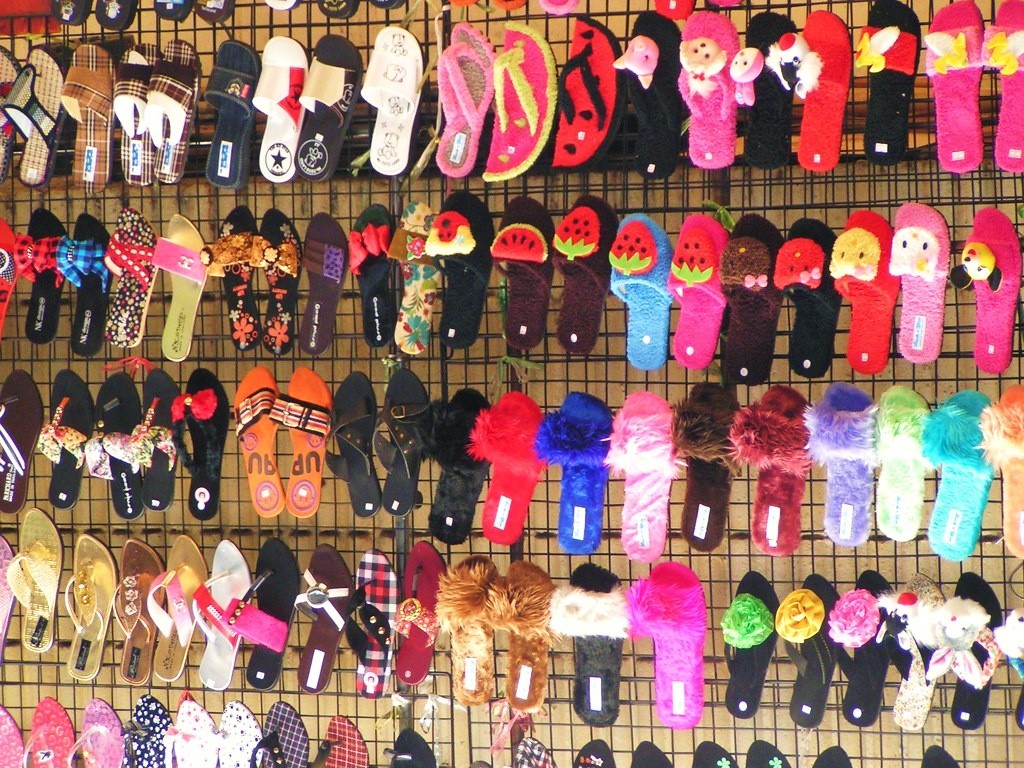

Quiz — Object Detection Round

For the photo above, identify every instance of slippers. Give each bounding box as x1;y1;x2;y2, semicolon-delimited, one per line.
0;0;1024;768
